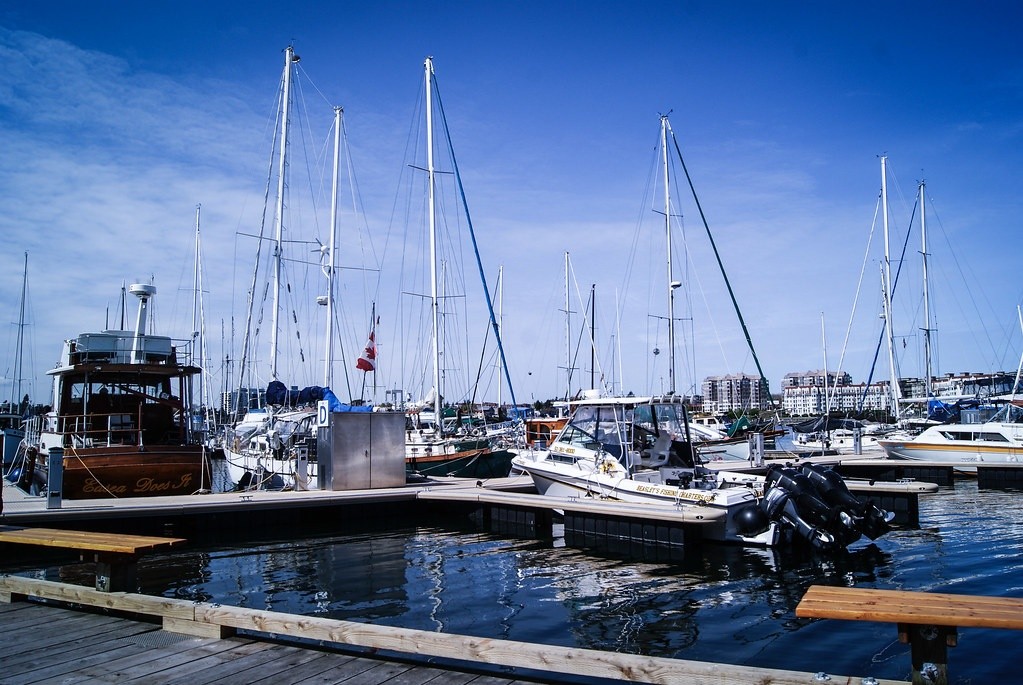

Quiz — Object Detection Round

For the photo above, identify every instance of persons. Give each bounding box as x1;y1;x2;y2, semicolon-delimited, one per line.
95;388;113;414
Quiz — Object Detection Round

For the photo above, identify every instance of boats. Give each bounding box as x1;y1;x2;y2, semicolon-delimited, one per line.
0;39;1022;579
